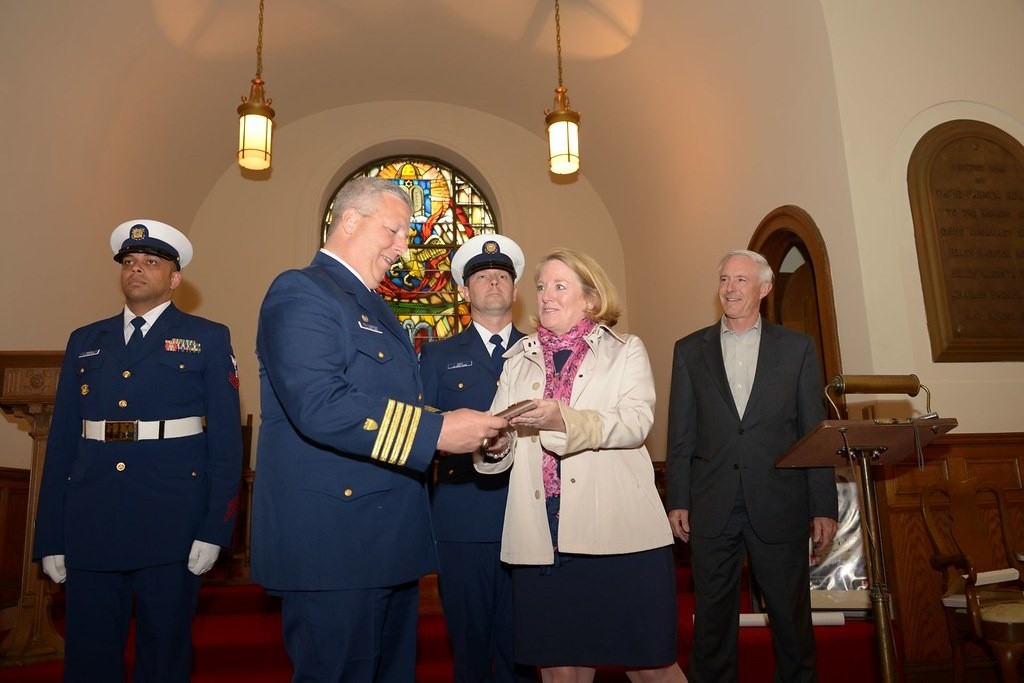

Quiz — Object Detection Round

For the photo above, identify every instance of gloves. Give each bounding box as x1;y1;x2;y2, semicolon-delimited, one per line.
187;540;220;575
42;555;67;584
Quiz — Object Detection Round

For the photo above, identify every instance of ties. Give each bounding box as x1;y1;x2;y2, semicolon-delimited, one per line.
127;316;147;353
489;334;506;374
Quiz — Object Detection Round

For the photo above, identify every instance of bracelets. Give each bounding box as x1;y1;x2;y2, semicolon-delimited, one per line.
486;433;511;459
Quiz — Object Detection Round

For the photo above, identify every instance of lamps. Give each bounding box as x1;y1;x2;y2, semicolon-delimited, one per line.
541;0;583;174
236;0;276;171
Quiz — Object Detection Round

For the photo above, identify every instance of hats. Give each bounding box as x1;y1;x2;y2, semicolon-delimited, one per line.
110;220;193;272
450;234;525;290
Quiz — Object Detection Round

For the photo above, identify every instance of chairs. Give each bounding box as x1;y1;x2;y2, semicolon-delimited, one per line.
919;479;1024;683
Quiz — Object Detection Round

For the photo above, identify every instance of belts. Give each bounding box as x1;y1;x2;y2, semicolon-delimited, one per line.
81;416;203;443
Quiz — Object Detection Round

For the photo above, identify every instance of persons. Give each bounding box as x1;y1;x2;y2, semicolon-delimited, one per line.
473;247;690;683
249;176;505;683
418;232;540;683
663;248;838;683
29;219;245;683
